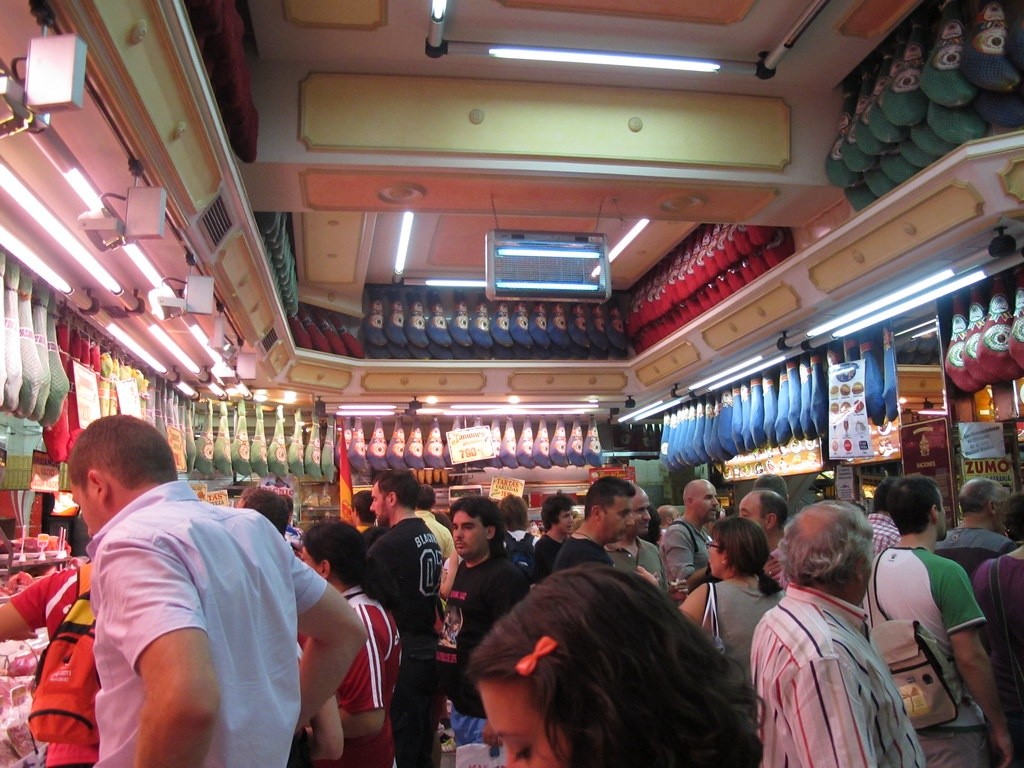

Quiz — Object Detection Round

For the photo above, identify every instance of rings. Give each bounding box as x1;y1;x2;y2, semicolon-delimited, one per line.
769;570;772;575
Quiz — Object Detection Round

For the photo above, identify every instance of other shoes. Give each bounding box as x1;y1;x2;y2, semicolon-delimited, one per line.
439;731;456;752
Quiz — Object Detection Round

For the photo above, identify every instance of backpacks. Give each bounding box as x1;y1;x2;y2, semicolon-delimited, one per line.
28;561;102;747
504;531;536;583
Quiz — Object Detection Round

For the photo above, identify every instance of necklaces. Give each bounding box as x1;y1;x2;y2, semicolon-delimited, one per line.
574;531;591;540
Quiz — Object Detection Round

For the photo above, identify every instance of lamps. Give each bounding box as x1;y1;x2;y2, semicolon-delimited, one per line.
617;224;1024;426
0;77;254;401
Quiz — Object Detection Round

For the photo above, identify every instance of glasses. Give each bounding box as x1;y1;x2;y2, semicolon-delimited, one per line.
705;540;720;550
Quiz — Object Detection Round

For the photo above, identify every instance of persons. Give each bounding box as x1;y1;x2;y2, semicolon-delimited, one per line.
658;475;1024;768
438;470;688;750
468;565;765;768
0;469;454;768
751;501;926;768
67;416;369;768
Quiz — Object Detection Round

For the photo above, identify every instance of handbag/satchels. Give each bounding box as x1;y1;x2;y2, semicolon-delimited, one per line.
868;618;959;728
455;743;505;768
